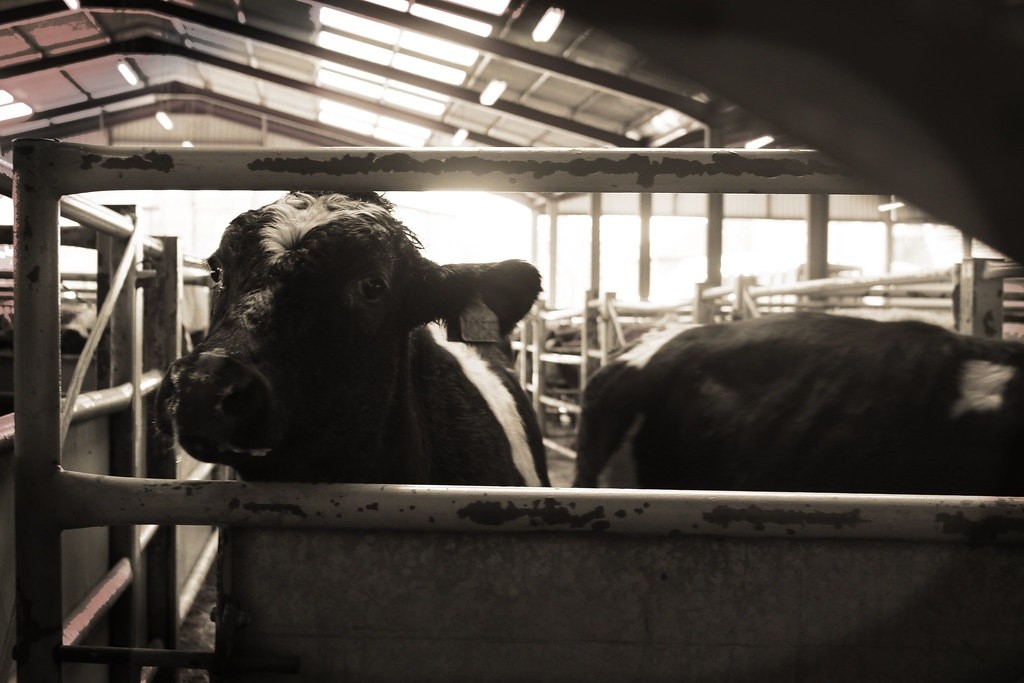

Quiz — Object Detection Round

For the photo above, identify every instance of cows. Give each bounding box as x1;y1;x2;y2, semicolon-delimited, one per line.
569;312;1024;496
152;190;550;488
